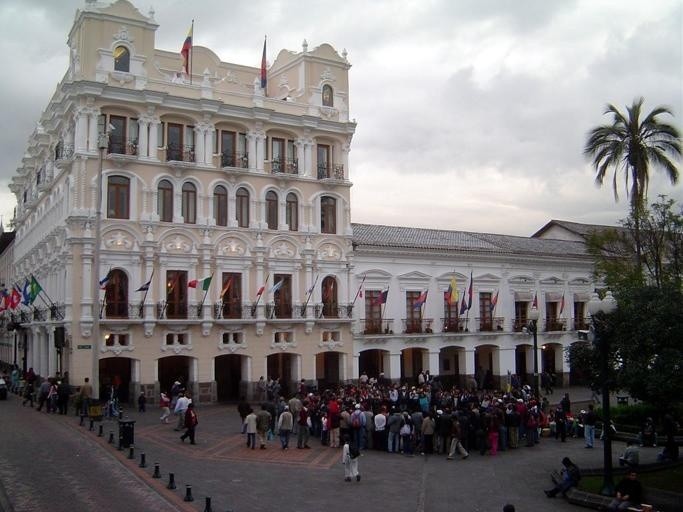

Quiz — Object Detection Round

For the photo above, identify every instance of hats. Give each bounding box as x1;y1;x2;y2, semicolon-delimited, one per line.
437;410;443;415
517;399;523;403
175;381;181;386
355;404;361;409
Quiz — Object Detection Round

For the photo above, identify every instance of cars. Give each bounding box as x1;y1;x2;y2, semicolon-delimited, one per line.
0;375;7;397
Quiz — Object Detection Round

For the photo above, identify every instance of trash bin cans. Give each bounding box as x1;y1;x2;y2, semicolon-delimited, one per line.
617;396;629;406
120;419;136;448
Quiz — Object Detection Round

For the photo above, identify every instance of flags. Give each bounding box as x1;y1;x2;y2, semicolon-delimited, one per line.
490;294;497;309
561;296;564;314
460;273;472;314
260;43;267;87
169;277;174;294
359;287;363;297
412;290;427;309
256;287;264;295
219;280;230;297
268;281;282;291
181;25;191;73
325;283;333;302
136;282;149;291
377;289;388;304
0;276;41;312
188;277;211;290
445;275;458;305
99;272;109;290
305;286;313;295
532;295;537;308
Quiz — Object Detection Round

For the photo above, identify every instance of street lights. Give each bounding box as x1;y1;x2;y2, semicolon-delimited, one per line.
586;287;618;497
528;304;541;411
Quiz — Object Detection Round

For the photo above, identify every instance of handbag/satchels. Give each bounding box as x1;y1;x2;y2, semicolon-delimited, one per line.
240;424;247;434
349;443;360;459
400;424;411;436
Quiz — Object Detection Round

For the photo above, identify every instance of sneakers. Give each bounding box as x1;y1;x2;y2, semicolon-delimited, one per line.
260;442;267;449
653;443;657;448
639;444;644;447
585;445;593;449
544;490;553;498
359;439;540;461
22;400;88;416
174;428;180;431
298;439;340;449
190;441;196;445
356;475;360;481
283;444;288;449
180;436;184;442
344;477;351;482
247;442;250;447
160;417;164;425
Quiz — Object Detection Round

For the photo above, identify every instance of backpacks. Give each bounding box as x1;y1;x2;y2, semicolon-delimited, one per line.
351;413;362;428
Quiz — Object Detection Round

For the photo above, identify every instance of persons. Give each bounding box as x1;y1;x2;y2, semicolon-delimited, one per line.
137;392;146;413
1;366;120;417
236;369;570;462
340;434;360;481
543;404;682;511
159;376;198;446
